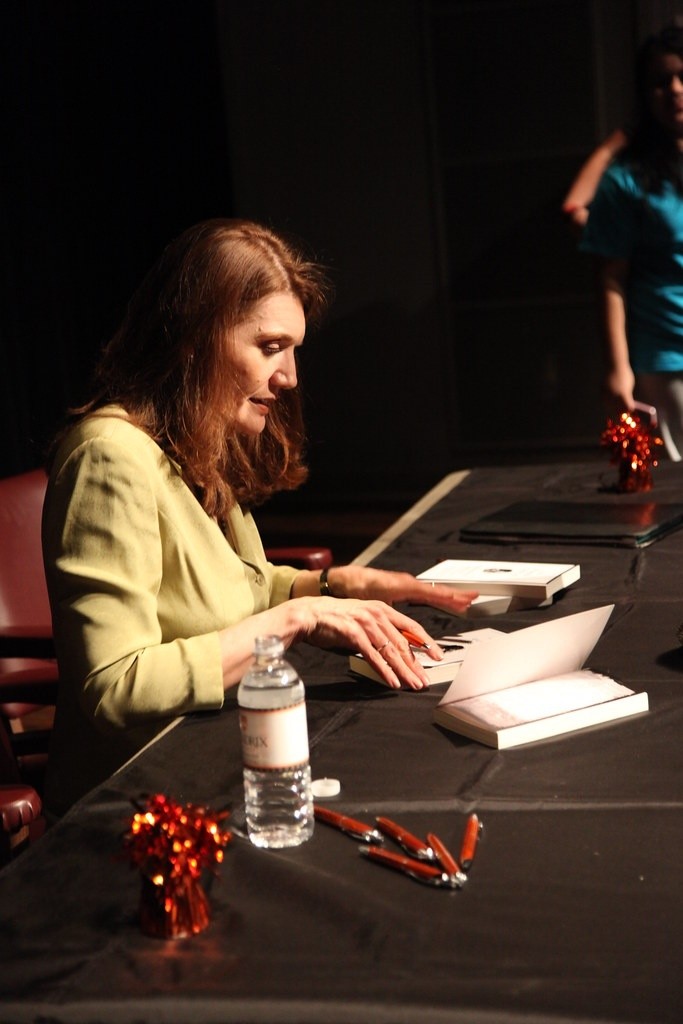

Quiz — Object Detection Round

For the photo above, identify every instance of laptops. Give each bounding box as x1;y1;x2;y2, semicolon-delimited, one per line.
459;501;683;548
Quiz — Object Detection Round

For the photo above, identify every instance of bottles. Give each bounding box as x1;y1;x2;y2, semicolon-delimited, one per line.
237;635;314;849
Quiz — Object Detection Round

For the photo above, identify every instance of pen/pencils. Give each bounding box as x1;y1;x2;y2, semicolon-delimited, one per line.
427;828;469;888
359;844;449;886
375;815;434;862
398;627;431;652
312;795;387;845
459;812;484;871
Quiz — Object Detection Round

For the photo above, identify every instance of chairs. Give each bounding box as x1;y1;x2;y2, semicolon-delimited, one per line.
0;468;332;875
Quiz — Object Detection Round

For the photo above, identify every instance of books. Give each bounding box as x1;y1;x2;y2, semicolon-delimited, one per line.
416;559;580;618
349;628;508;689
434;604;648;750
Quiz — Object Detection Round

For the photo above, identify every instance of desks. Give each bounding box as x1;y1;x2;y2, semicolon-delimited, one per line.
0;446;683;1024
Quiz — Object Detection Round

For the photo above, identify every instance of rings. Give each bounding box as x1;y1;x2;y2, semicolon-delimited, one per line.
431;581;435;587
378;640;390;651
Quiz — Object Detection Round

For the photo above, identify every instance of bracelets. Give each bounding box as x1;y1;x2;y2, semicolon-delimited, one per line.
320;566;333;597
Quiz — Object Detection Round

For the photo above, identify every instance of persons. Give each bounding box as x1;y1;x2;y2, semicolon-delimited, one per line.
563;14;683;464
41;222;481;835
